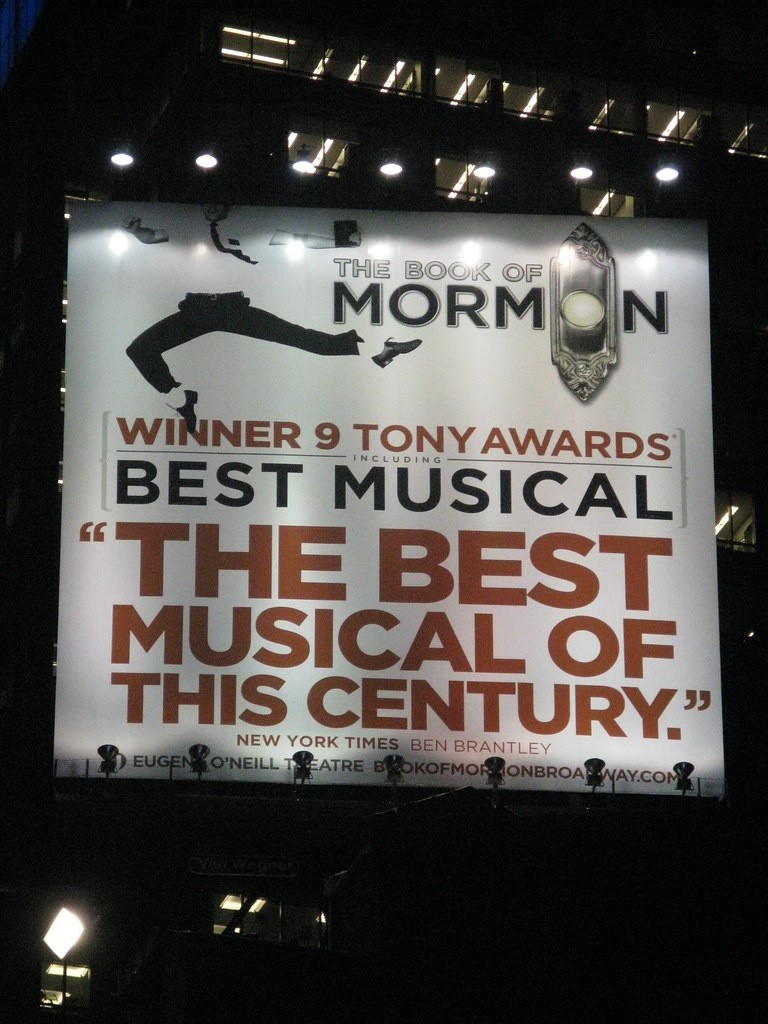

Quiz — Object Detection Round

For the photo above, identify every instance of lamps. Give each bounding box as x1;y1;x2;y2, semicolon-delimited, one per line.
673;761;694;795
383;754;405;786
189;744;211;781
292;751;314;784
484;757;506;789
96;744;119;778
584;758;606;793
292;143;315;172
